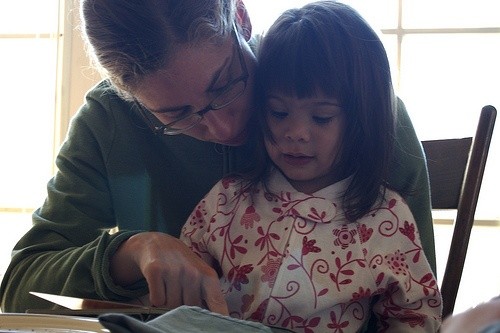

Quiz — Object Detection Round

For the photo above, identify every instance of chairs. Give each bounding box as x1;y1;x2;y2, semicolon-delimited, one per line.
421;105;497;322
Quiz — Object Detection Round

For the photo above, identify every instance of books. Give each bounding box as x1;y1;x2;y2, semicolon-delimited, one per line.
0;305;299;333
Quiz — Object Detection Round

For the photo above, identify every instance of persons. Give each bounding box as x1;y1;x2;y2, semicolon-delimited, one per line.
178;0;443;333
0;0;436;315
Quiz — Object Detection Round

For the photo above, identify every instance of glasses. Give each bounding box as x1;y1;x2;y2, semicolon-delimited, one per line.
133;19;249;136
